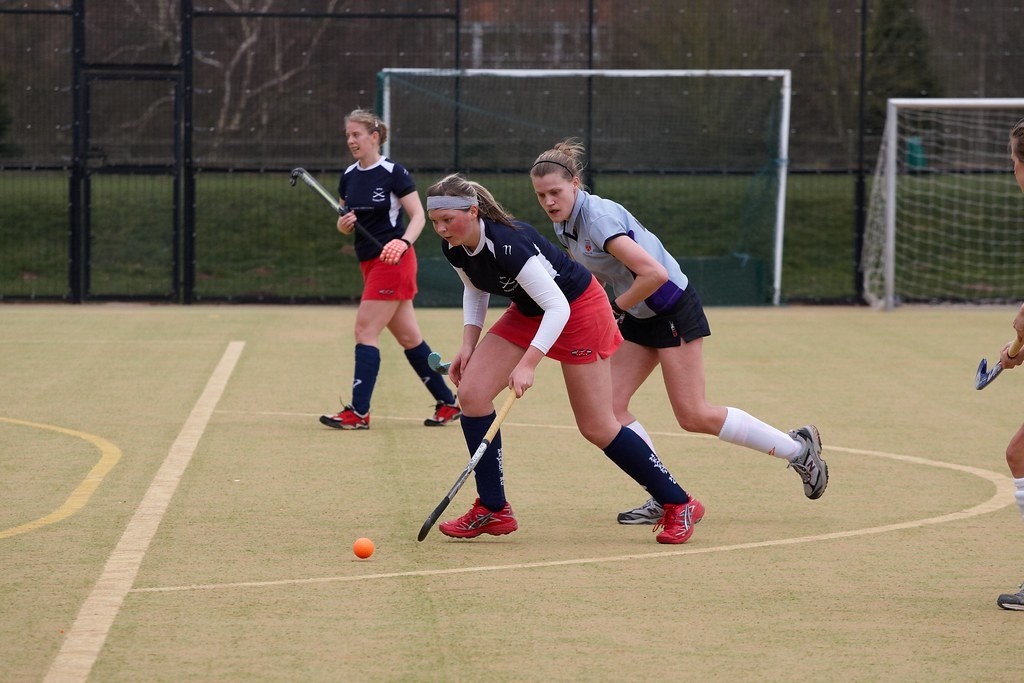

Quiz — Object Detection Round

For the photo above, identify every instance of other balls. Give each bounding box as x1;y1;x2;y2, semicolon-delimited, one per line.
352;536;376;560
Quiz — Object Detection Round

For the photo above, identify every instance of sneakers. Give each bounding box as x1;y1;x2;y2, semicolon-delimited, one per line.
424;394;463;426
997;582;1024;611
653;493;705;544
617;496;664;525
786;424;829;499
320;393;369;430
439;498;518;539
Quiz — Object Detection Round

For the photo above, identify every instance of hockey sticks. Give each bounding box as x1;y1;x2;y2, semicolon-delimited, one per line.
417;390;515;542
290;167;399;264
974;332;1024;391
427;351;453;375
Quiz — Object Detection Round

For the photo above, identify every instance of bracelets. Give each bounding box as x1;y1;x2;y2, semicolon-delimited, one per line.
610;300;624;314
399;238;410;248
614;312;621;319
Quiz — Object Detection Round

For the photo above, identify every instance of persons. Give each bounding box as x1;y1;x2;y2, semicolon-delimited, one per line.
997;118;1024;612
320;108;828;545
